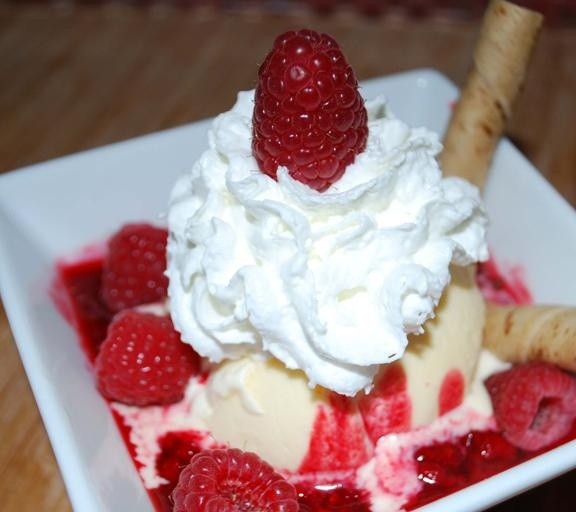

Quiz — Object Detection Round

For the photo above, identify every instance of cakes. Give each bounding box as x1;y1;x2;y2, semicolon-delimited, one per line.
67;0;576;512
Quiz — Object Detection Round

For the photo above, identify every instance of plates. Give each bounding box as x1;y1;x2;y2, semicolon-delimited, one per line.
1;64;575;512
